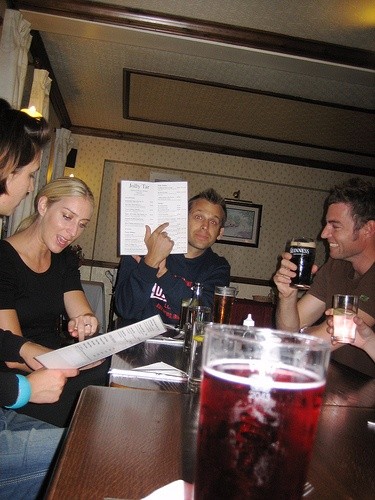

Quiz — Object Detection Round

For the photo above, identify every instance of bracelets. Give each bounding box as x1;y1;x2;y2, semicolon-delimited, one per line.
5;374;31;409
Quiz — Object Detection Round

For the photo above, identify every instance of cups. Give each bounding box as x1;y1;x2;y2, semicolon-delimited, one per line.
331;293;359;343
289;236;317;289
187;322;212;394
194;321;331;500
213;286;236;325
182;305;212;354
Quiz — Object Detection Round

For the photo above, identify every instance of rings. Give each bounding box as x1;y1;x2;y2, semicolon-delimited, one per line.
85;324;92;327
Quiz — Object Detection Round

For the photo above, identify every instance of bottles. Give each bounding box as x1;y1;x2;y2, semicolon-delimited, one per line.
241;313;255;352
185;281;203;348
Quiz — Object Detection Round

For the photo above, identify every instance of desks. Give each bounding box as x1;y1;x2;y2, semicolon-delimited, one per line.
40;318;375;500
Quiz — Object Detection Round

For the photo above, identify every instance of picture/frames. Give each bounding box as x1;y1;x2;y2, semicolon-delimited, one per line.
214;198;262;248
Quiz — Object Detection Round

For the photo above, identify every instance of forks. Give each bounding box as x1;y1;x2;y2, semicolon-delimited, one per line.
303;482;314;498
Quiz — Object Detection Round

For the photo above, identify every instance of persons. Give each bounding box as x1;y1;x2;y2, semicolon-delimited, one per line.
273;177;375;378
0;176;111;427
113;189;231;364
0;97;80;500
325;308;375;363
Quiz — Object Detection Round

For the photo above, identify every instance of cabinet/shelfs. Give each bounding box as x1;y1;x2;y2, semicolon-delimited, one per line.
229;298;274;328
